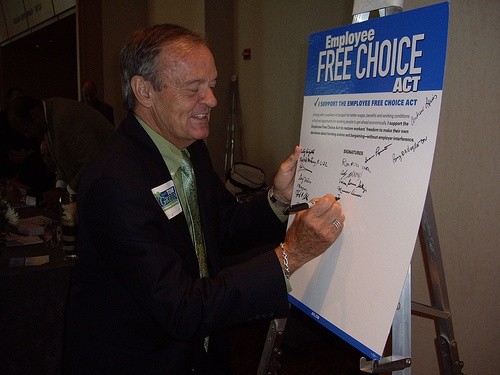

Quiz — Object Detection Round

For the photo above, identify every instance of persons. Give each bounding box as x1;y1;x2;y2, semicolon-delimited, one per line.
0;95;116;202
80;79;115;127
59;23;345;375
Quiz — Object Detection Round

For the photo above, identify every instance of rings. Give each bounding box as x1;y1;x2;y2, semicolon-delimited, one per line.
333;219;342;230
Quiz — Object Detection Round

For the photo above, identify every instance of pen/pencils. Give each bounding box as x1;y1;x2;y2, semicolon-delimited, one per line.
288;197;341;212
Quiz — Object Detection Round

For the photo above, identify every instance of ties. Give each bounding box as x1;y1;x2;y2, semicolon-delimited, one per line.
180;149;211;354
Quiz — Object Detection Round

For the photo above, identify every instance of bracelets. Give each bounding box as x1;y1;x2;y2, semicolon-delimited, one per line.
269;185;291;208
280;242;291;280
